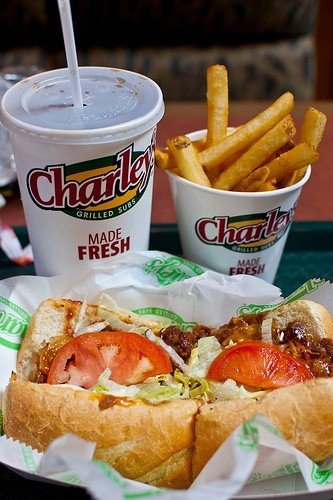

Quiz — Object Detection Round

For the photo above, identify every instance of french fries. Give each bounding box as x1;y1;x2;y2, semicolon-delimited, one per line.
156;63;326;192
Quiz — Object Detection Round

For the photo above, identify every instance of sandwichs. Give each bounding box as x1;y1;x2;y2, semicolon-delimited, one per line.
2;296;333;490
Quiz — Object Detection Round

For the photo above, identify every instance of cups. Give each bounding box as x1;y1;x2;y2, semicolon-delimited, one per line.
0;66;165;278
167;128;312;285
